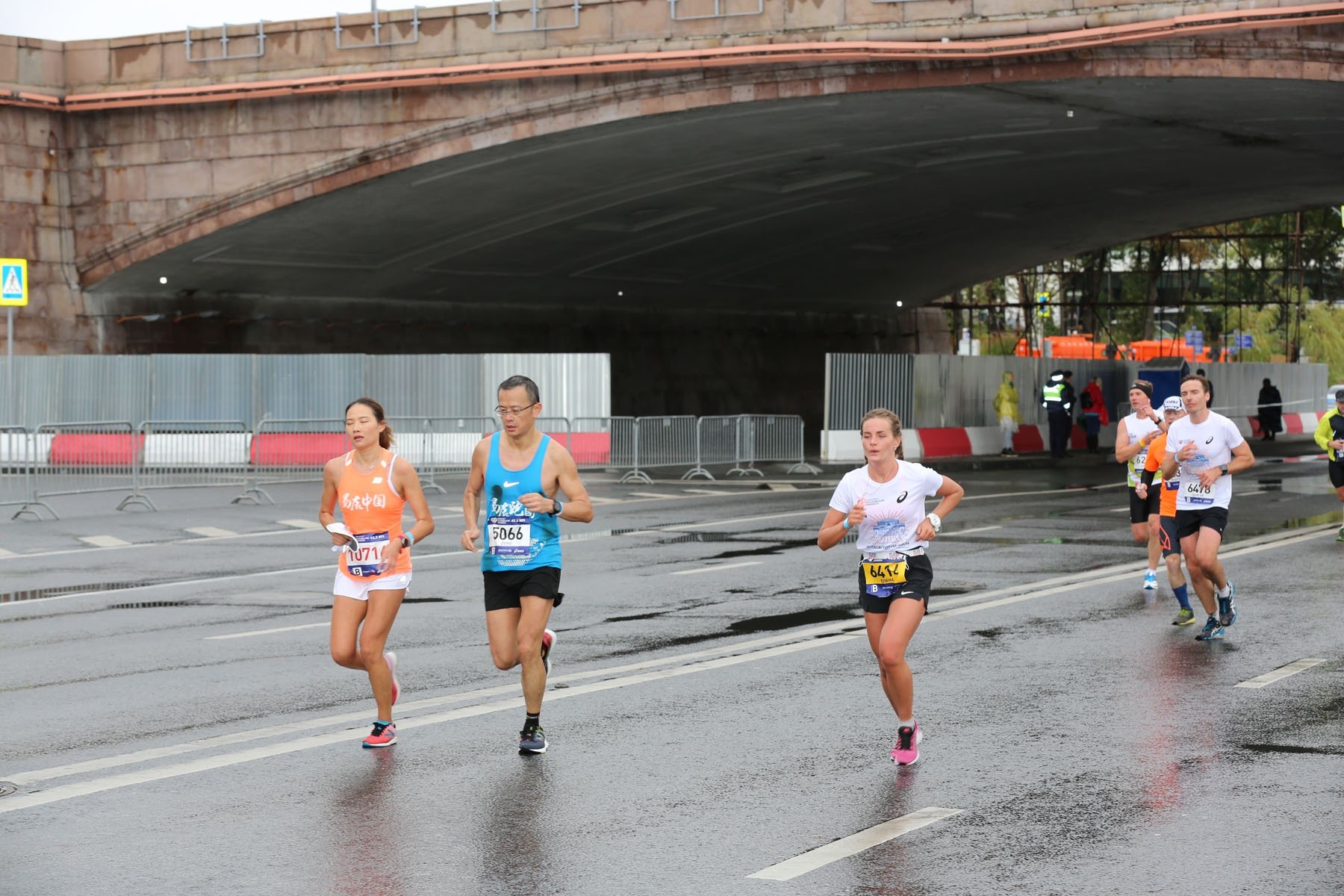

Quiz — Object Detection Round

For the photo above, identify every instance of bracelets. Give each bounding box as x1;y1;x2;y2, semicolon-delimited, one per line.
405;531;414;545
843;517;852;531
1154;417;1163;425
555;501;564;516
1174;454;1182;463
1139;438;1146;448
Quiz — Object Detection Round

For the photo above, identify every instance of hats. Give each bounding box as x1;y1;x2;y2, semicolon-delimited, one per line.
1130;379;1153;400
1163;395;1187;413
1335;389;1344;402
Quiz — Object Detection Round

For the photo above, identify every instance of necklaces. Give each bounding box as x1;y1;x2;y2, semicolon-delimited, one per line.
879;462;895;486
369;465;373;469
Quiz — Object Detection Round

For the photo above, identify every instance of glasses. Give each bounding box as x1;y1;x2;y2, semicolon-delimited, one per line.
494;403;537;416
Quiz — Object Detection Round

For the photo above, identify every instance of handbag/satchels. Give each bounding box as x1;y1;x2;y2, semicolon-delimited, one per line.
1099;405;1110;426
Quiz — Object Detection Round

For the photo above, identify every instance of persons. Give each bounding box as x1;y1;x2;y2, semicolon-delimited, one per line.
462;375;593;755
1135;368;1214;626
1314;389;1344;541
1115;379;1168;590
1258;378;1283;441
993;370;1109;458
319;397;434;747
1163;375;1255;641
818;407;965;765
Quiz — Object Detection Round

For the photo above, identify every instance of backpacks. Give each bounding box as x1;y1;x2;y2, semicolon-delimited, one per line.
1080;389;1093;409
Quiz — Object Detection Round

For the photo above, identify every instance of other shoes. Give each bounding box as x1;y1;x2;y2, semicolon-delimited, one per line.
1261;436;1276;442
1335;528;1344;541
1001;448;1018;458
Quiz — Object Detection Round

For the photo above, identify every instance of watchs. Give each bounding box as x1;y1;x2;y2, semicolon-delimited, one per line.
926;513;941;532
548;500;562;518
1219;465;1228;476
397;535;408;548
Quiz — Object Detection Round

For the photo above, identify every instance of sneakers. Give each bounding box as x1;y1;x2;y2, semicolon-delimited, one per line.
1143;569;1159;589
1216;581;1238;626
1171;608;1196;626
1195;617;1227;640
517;724;549;755
895;720;920;765
539;628;557;678
362;721;398;747
890;730;923;760
382;651;401;706
